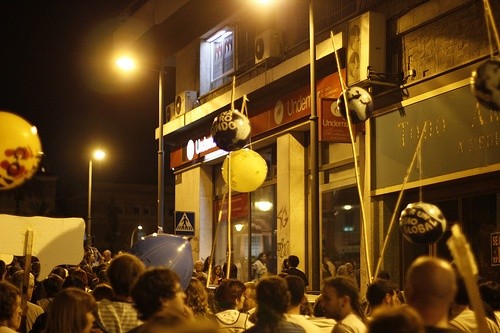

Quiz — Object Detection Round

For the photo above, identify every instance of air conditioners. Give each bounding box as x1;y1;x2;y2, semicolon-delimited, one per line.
165;102;175;123
254;29;281;66
175;91;197;118
346;11;386;88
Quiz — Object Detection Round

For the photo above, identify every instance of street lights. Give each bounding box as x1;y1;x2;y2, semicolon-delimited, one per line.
114;55;171;234
87;149;106;247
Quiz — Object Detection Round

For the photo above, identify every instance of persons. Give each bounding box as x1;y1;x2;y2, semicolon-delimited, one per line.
278;255;309;291
214;262;500;333
322;255;359;284
230;253;278;280
0;249;214;333
404;255;462;333
